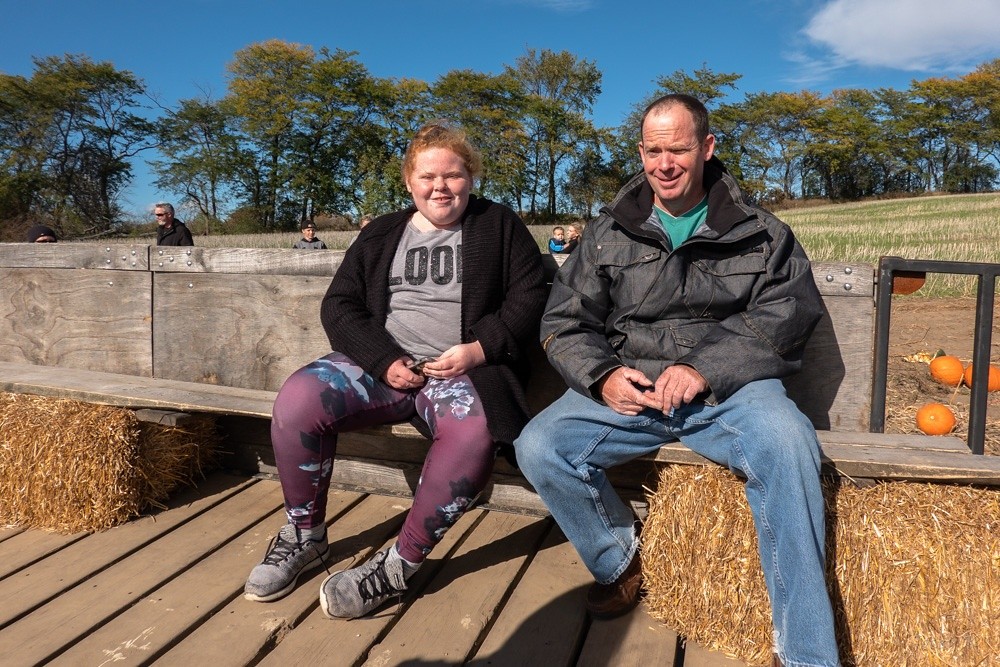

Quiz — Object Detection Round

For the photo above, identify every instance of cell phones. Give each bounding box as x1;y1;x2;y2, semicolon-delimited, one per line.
405;357;437;374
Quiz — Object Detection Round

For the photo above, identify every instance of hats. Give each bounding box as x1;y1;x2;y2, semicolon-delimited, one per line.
28;226;57;243
301;220;317;228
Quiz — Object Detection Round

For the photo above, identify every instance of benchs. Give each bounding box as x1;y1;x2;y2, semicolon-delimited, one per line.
0;239;1000;521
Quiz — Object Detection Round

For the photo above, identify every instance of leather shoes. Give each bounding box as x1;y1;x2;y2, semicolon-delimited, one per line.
586;550;641;617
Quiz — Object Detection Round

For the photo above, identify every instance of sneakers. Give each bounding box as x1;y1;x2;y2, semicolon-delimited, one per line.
244;524;330;602
320;548;408;619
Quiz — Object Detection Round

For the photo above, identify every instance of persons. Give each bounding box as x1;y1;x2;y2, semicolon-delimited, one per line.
29;226;57;243
155;203;194;246
243;126;559;620
562;222;584;254
512;94;843;667
548;226;570;254
349;216;372;249
293;220;328;250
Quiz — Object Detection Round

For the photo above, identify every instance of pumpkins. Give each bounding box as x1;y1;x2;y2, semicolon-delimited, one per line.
964;362;1000;392
930;356;965;387
916;403;957;435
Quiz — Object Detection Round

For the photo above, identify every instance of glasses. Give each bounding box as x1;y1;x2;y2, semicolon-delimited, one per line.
156;212;168;216
36;240;56;243
